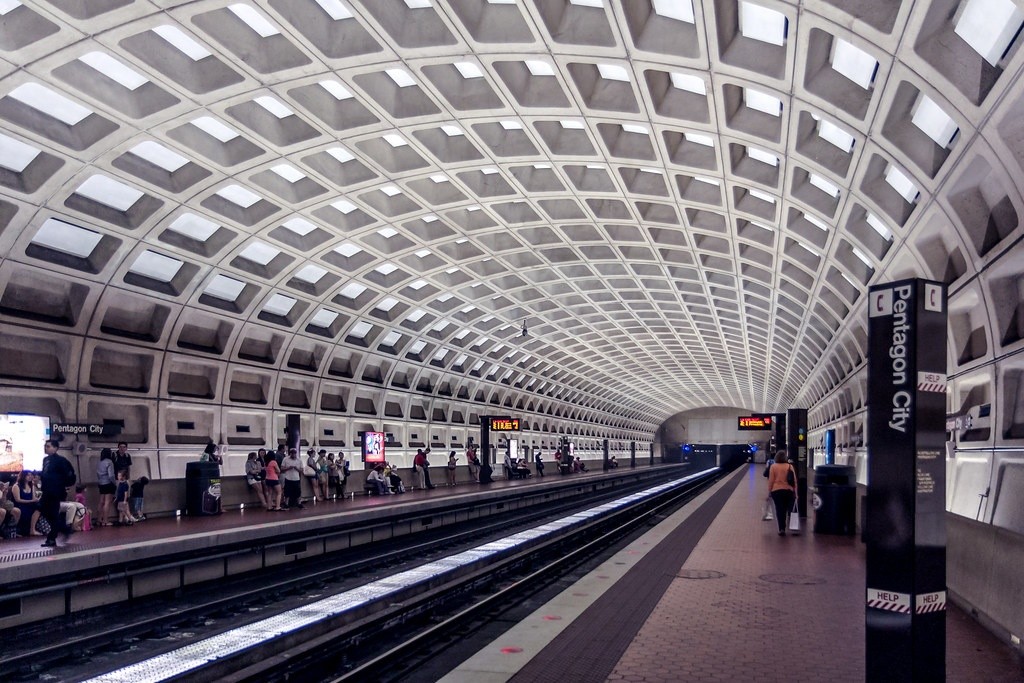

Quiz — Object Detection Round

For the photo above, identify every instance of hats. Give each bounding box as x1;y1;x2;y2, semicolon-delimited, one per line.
289;448;297;453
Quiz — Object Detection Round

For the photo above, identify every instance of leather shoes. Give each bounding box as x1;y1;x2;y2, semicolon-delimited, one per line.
62;524;72;541
41;541;57;547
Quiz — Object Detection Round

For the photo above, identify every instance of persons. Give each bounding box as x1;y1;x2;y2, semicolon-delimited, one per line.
504;451;532;480
467;445;495;484
535;452;545;477
414;448;436;490
200;443;350;511
763;450;799;536
555;449;589;474
609;457;618;469
0;439;149;547
448;450;460;486
367;461;406;496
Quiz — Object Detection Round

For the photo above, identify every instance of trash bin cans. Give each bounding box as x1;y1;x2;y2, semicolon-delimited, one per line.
813;464;858;536
185;462;222;516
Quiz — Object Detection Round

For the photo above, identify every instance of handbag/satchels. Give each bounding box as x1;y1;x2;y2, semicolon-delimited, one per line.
760;497;775;521
787;464;795;486
789;499;801;530
303;466;316;476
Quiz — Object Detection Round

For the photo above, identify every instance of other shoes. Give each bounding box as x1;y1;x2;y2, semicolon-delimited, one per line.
298;504;307;509
97;516;146;526
274;507;282;511
30;531;43;536
282;505;289;510
267;507;275;511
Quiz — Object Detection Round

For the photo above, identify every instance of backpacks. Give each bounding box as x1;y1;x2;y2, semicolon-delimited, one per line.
63;461;76;486
